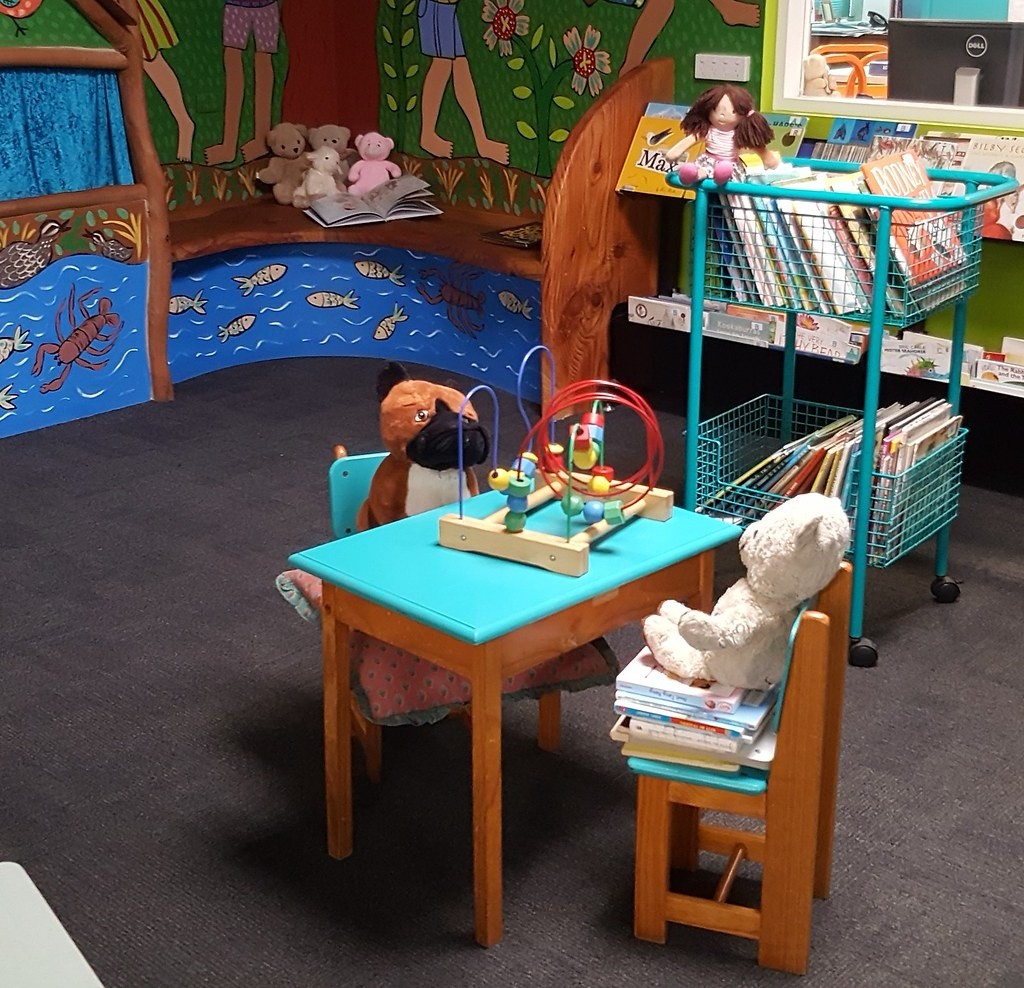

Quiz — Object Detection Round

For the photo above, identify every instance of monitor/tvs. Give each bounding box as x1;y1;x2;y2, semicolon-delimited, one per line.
888;18;1024;108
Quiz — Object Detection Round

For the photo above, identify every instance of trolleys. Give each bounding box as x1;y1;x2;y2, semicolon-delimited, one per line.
665;156;1020;667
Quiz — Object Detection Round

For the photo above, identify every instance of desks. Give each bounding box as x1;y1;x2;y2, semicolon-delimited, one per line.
285;470;741;951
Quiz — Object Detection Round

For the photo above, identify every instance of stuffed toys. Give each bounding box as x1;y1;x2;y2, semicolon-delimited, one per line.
804;55;840;99
640;489;856;685
664;85;777;184
357;361;487;533
256;120;403;209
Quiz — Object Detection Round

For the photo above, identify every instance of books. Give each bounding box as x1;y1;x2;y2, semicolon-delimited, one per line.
617;101;705;201
709;150;966;324
302;171;444;228
696;394;967;556
607;645;791;758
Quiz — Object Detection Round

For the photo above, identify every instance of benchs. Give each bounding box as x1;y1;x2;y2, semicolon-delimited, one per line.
153;190;562;415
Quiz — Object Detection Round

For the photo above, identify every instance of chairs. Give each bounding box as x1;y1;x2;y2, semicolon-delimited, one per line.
331;439;562;785
628;557;852;977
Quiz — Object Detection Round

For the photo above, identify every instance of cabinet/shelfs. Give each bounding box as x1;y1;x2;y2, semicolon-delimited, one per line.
664;150;1019;668
